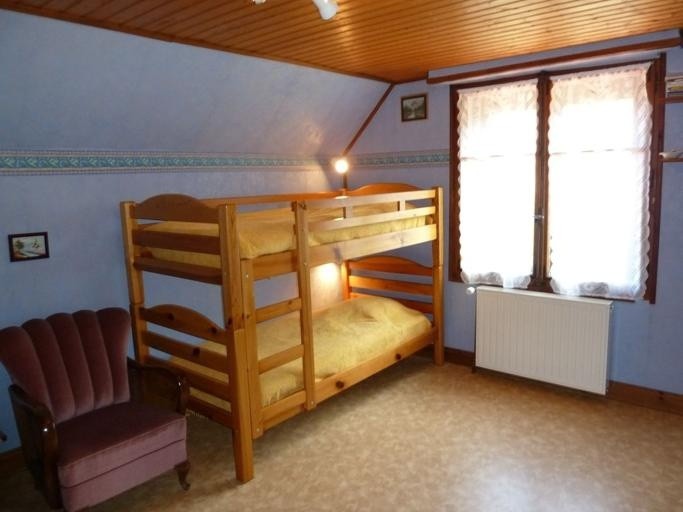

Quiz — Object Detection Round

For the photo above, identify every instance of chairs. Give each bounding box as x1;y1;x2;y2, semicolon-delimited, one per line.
0;306;194;511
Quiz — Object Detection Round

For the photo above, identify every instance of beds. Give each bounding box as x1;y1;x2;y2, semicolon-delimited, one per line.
118;180;446;485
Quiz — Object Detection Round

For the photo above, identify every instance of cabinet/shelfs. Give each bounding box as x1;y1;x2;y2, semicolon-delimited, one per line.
653;94;682;163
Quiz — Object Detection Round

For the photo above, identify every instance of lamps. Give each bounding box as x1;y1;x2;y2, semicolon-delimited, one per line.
251;0;340;21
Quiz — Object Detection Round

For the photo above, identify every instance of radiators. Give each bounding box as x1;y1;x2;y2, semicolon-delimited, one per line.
473;284;616;398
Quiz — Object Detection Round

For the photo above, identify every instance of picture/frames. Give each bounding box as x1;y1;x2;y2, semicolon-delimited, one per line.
7;231;51;263
399;91;429;122
